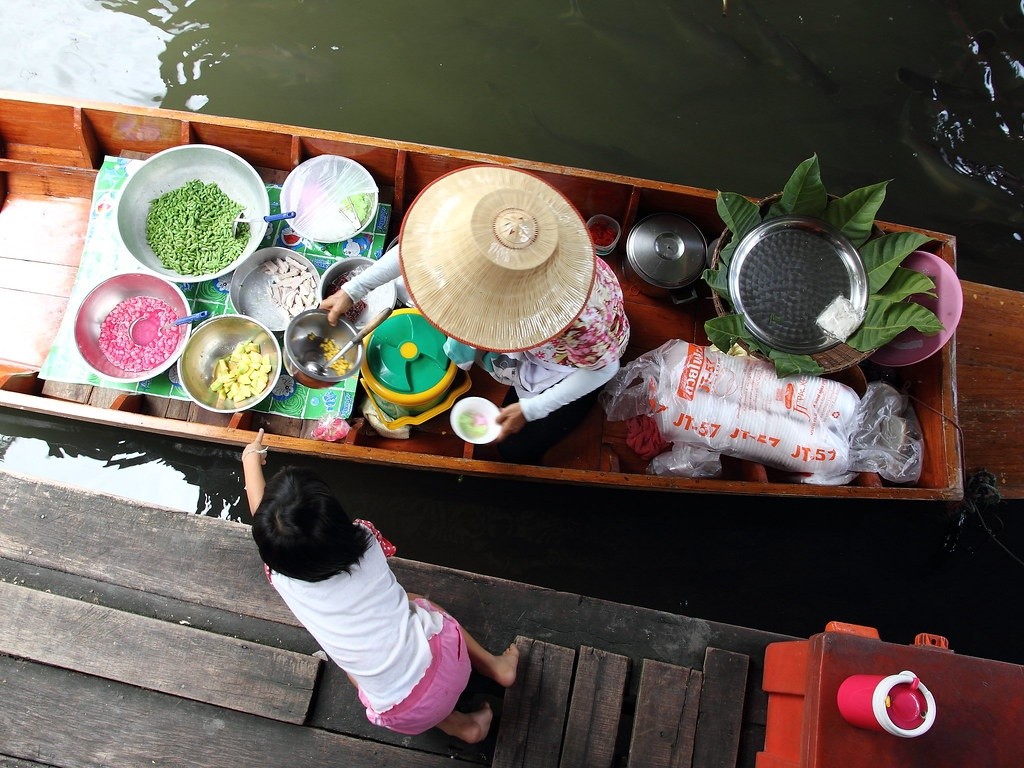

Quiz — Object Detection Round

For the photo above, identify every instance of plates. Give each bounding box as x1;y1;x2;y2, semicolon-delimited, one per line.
449;396;503;444
280;156;379;243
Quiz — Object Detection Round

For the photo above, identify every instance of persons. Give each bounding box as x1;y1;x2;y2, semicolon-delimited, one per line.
242;429;519;742
318;165;629;443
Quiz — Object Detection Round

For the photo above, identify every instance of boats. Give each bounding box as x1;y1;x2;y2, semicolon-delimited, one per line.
0;91;1024;509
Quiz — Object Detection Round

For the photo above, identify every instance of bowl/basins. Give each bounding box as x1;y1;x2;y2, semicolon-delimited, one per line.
585;215;620;255
117;145;270;284
74;272;191;383
176;314;283;413
229;248;321;332
871;251;963;368
320;258;397;329
654;341;859;473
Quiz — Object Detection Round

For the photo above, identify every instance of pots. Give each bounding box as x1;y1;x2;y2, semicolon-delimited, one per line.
282;310;364;388
624;211;708;306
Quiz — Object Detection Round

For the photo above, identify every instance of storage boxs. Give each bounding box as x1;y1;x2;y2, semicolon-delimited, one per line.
756;621;1024;768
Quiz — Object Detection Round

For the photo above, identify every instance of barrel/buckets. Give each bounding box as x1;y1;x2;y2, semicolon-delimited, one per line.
360;307;472;430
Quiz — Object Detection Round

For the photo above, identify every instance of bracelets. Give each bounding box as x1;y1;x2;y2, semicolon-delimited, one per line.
242;447;269;459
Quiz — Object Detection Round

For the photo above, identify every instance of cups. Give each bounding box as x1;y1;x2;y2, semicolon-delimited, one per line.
837;672;936;739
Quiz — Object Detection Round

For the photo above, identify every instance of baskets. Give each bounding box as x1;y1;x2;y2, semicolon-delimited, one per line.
710;191;907;375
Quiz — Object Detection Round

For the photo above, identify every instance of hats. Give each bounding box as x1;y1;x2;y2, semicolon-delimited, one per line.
397;165;597;353
